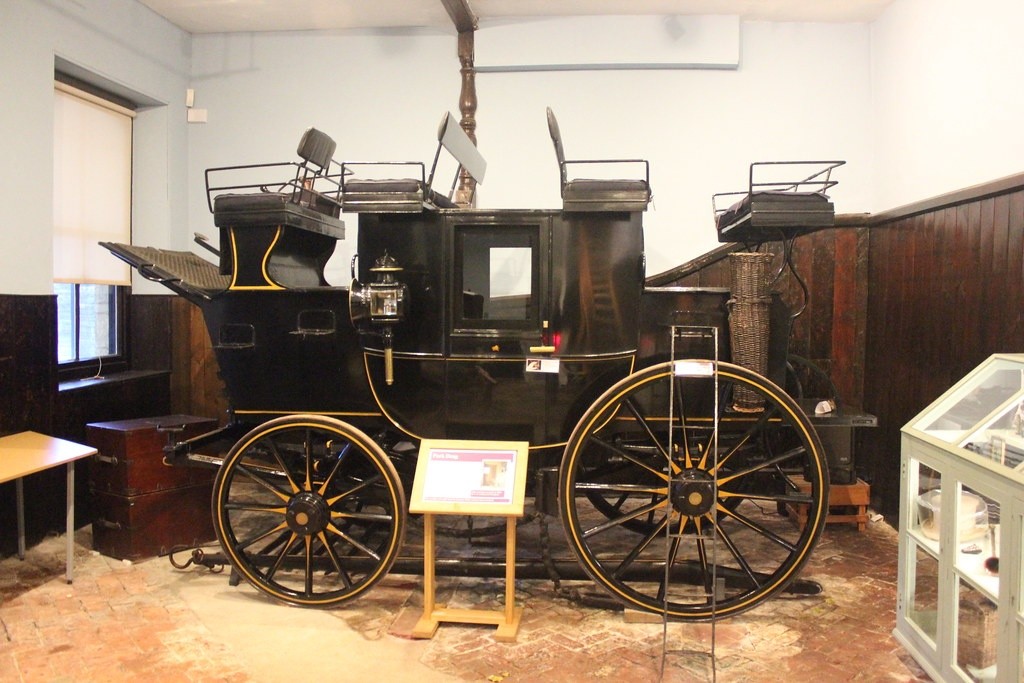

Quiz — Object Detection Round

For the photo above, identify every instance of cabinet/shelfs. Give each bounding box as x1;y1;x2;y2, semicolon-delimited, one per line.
900;352;1024;683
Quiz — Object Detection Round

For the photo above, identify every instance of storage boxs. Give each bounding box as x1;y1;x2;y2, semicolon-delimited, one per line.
917;487;989;541
89;484;218;565
86;413;220;494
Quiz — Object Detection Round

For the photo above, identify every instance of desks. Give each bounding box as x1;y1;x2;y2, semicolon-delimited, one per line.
0;429;99;584
786;474;871;532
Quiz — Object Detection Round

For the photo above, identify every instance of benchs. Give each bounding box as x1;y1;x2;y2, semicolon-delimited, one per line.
339;110;486;213
204;128;347;285
712;153;845;315
544;103;654;213
